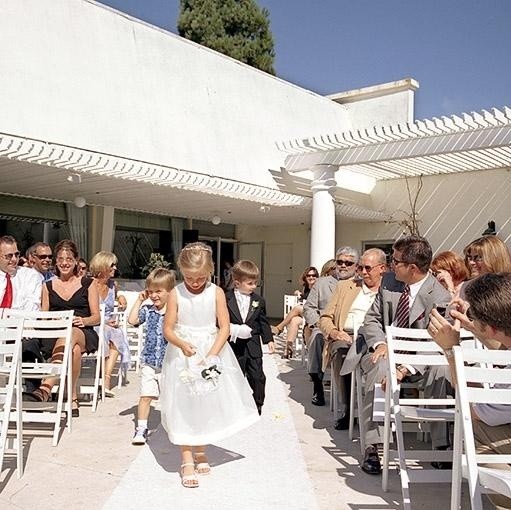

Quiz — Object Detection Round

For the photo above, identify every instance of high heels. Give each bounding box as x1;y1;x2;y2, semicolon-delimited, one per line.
272;328;284;336
282;350;294;359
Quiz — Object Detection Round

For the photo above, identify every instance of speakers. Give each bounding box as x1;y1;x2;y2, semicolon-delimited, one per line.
182;230;198;248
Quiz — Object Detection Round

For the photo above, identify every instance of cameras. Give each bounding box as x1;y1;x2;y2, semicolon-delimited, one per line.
433;303;456;325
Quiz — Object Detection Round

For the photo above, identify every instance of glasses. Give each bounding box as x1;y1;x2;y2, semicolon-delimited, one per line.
306;273;317;277
336;259;356;267
36;254;53;260
358;264;383;271
465;254;484;263
1;251;20;259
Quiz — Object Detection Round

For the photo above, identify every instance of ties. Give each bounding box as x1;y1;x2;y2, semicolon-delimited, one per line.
396;285;411;365
0;273;13;310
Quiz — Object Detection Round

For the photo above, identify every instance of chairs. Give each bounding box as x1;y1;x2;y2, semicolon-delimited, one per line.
324;316;385;455
382;325;511;510
283;295;311;370
1;289;155;488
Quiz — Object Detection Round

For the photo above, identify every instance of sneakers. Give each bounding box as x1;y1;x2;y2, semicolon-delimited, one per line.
132;427;150;445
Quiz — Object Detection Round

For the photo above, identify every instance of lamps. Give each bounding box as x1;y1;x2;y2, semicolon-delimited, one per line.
74;195;87;209
209;211;222;226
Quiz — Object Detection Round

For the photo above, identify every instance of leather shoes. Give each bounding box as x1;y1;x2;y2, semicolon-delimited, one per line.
431;447;453;470
333;415;356;431
310;385;326;408
361;444;382;476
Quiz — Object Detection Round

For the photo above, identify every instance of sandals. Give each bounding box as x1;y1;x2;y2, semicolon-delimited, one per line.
194;452;212;476
68;399;80;417
33;383;53;402
180;463;200;488
103;375;115;397
